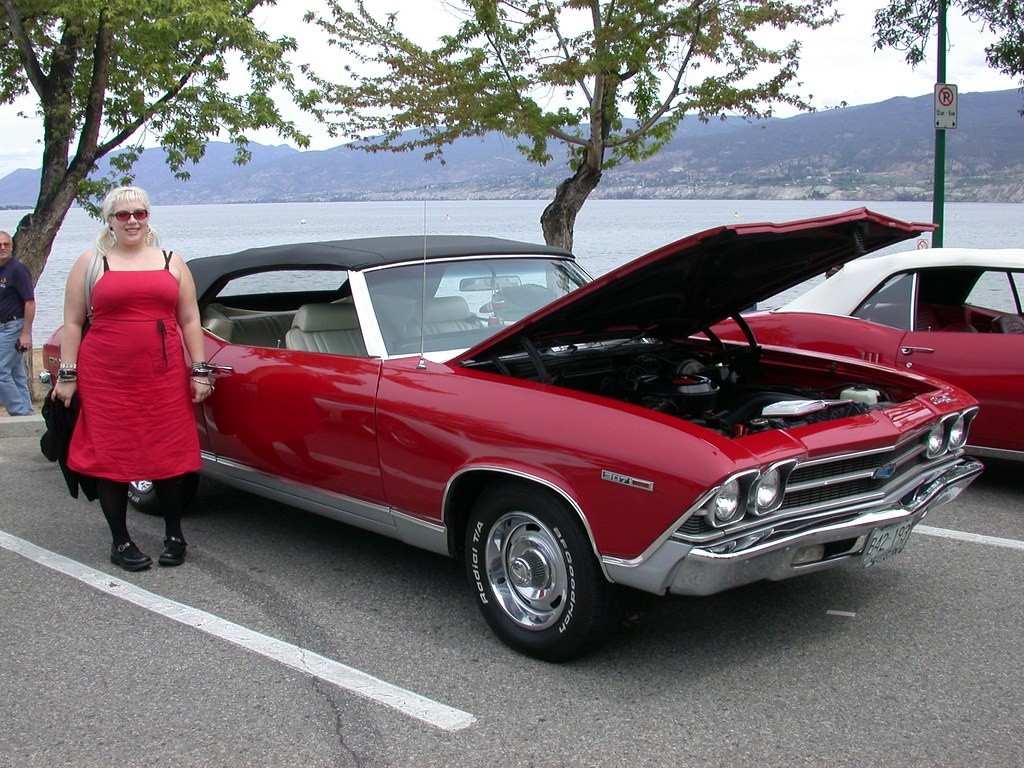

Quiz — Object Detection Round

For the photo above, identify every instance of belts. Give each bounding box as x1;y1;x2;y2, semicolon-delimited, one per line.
0;314;24;323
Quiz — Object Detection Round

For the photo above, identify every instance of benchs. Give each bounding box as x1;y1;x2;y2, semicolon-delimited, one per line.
204;310;298;346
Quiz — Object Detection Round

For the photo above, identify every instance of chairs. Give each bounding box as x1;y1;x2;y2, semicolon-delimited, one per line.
404;296;484;336
878;294;1024;334
285;304;371;357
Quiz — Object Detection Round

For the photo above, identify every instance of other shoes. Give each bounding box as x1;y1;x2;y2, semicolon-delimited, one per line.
158;535;188;565
110;541;153;571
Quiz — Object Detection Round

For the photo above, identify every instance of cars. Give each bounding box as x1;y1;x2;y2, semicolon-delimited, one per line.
690;247;1024;462
37;206;984;665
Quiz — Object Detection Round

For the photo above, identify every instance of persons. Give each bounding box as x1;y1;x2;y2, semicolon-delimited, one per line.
0;231;35;416
51;187;212;573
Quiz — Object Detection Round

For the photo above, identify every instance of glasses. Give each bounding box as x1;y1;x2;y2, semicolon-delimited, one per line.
0;243;10;247
110;210;148;221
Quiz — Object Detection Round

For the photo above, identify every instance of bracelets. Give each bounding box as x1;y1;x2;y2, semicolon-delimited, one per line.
190;362;211;385
57;364;77;382
